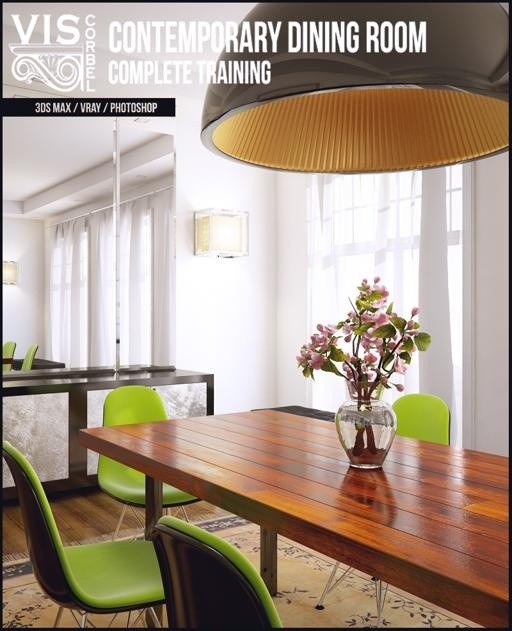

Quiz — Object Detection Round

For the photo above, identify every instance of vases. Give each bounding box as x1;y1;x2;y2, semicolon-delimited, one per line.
336;376;396;468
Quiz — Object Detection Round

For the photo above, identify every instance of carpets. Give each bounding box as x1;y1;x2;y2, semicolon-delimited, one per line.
2;512;483;631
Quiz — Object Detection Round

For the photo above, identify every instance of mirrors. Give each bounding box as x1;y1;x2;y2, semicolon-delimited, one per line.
3;85;174;377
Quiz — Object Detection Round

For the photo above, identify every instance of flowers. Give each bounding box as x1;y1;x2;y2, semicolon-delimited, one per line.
298;277;431;393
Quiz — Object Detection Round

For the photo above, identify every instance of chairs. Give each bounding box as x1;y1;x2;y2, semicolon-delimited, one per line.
153;515;283;628
2;340;17;372
1;441;164;630
316;393;450;619
98;385;191;540
21;344;38;371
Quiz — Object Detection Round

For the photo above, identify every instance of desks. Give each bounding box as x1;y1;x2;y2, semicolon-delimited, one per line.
78;409;510;628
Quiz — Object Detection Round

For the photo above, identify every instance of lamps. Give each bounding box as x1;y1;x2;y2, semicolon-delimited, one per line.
194;208;249;260
199;4;508;174
3;261;17;286
